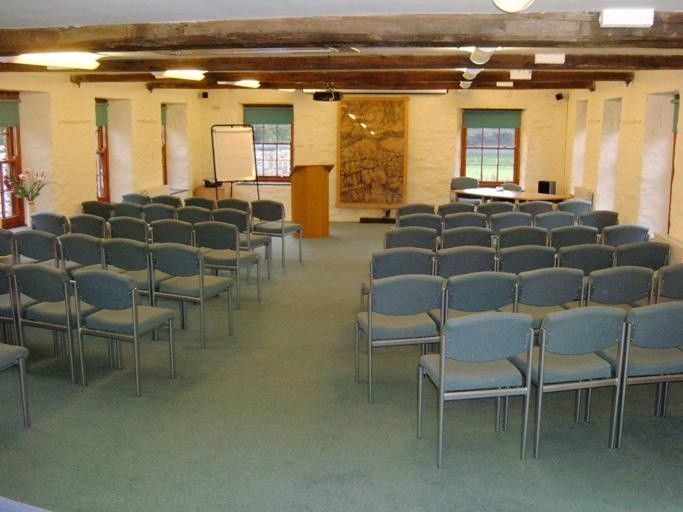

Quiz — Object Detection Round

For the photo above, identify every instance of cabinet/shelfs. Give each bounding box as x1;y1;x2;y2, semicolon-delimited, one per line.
192;186;225;202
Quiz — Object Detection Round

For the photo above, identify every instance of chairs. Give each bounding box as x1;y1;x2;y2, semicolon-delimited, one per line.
450;176;479;201
500;183;523;192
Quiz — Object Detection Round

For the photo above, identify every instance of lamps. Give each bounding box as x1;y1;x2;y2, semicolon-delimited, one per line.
459;0;566;89
599;7;655;28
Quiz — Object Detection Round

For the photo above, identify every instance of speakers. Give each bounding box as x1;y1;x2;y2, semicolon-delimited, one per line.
555;93;563;100
202;91;209;99
538;180;556;194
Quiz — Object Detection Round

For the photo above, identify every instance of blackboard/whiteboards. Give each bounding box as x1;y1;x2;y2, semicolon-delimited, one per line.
211;124;258;182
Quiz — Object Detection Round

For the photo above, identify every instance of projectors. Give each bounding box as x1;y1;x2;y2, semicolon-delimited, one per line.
313;91;343;102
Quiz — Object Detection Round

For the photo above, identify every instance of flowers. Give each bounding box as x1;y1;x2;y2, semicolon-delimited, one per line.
2;168;50;200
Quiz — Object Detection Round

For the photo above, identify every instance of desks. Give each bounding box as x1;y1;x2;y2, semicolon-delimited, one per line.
449;187;573;205
